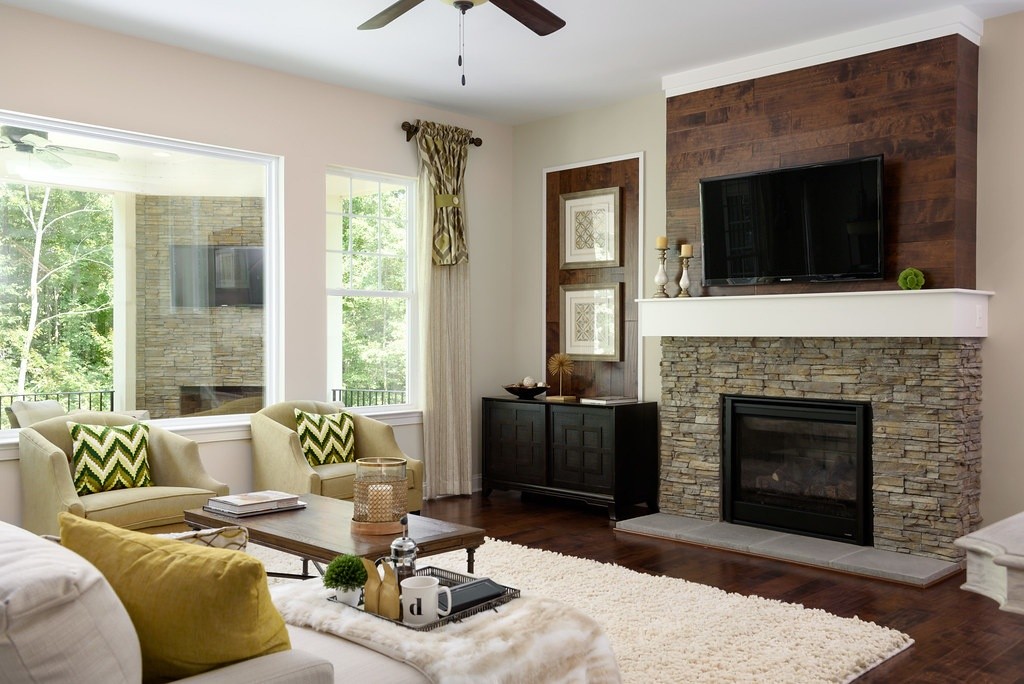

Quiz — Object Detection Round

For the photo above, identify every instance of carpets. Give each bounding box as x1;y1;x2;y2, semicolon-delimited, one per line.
241;537;916;684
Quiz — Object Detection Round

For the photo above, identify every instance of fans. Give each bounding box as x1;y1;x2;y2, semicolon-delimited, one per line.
357;0;566;37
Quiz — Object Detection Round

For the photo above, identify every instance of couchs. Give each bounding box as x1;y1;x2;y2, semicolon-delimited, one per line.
166;621;436;684
19;409;230;535
250;400;424;516
6;397;150;427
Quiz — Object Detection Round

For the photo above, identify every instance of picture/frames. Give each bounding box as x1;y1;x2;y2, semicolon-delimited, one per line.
559;282;621;362
559;186;621;270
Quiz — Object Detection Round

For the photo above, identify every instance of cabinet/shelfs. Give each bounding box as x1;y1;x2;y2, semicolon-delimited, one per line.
481;396;660;522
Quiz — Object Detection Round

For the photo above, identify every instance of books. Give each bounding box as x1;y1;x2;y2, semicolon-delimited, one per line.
207;490;299;515
202;501;308;518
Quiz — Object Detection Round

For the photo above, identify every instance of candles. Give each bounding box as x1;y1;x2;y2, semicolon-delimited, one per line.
657;235;668;248
366;484;392;523
681;244;693;257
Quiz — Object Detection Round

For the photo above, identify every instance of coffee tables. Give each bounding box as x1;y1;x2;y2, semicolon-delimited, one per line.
183;493;485;579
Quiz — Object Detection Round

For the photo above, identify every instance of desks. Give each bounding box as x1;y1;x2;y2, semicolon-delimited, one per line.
954;509;1024;615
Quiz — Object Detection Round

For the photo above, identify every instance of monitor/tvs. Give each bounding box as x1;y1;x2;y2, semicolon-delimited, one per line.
698;153;886;287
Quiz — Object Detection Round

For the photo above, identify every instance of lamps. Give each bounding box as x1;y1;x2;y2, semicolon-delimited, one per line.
441;0;487;85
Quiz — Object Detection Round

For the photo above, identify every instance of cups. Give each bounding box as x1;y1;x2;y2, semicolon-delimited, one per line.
400;575;452;627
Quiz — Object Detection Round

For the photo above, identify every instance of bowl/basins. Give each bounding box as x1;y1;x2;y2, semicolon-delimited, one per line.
503;384;550;396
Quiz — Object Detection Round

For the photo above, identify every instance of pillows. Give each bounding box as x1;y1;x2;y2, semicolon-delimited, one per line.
293;407;355;468
57;511;291;680
0;520;142;684
66;421;154;496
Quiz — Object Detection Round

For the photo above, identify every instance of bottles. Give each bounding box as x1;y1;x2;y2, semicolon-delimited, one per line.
375;537;418;593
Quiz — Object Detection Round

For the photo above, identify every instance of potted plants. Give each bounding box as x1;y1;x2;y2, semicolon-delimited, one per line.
323;555;368;607
